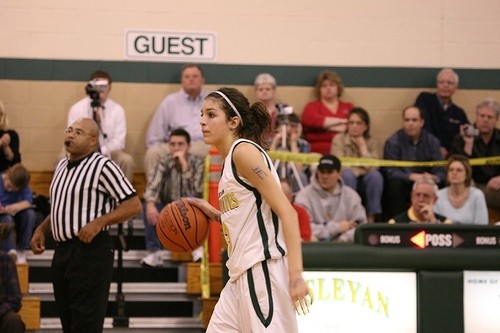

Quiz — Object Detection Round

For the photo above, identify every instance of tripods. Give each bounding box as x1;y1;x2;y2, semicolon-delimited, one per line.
273;124;304;189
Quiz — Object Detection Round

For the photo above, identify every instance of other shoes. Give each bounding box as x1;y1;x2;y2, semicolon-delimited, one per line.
139;251;164;268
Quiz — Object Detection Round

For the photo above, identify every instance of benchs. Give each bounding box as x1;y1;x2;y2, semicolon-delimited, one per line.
24;219;202;333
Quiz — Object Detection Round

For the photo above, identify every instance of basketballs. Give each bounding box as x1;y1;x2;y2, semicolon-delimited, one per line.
156;199;211;251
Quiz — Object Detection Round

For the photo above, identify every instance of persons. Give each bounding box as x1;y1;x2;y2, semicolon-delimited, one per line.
31;118;141;333
67;72;135;174
380;70;500;248
140;65;210;266
186;88;313;333
0;101;36;332
255;71;382;244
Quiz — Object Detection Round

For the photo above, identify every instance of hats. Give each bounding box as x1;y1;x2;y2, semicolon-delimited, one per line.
317;155;341;171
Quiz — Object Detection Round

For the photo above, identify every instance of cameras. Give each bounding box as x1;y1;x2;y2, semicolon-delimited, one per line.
86;81;109;92
465;124;480;137
